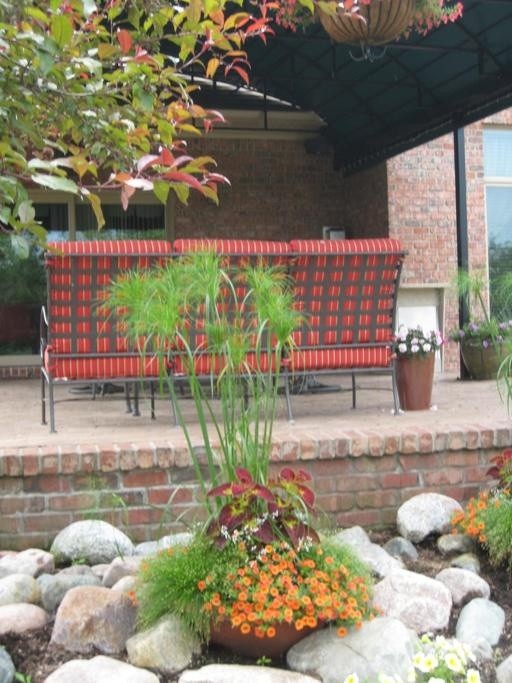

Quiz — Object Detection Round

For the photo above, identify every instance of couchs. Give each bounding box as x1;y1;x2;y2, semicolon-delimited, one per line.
37;234;408;435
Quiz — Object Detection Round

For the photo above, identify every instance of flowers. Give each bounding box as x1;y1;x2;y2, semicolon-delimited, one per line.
385;328;443;355
447;316;512;348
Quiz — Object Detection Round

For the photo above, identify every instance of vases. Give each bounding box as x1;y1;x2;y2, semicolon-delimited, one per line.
208;604;327;659
394;356;434;410
461;342;510;379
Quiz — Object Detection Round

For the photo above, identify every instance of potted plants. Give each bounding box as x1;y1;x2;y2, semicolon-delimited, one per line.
136;469;384;646
